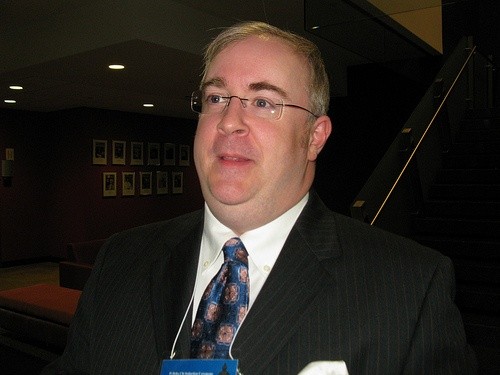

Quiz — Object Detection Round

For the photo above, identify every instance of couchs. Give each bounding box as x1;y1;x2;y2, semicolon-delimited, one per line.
52;237;106;289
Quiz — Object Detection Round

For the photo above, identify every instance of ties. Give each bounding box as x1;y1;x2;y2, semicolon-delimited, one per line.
186;237;251;359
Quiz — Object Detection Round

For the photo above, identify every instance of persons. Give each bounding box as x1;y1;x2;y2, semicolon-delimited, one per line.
55;22;476;375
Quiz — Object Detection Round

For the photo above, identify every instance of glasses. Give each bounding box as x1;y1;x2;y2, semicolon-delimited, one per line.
188;87;319;122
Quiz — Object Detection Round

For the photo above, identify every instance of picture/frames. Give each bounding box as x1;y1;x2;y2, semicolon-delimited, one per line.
92;139;107;166
179;144;190;167
156;170;169;195
122;171;136;196
163;143;175;167
130;141;144;167
139;171;153;196
148;142;161;167
171;170;184;195
101;170;118;197
111;139;126;166
